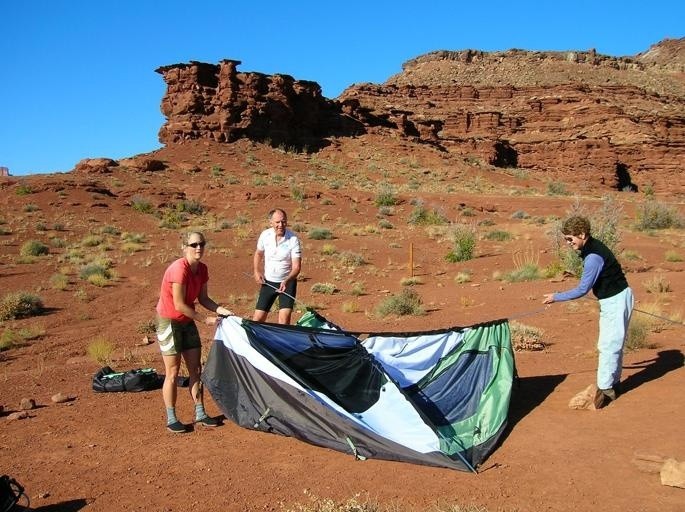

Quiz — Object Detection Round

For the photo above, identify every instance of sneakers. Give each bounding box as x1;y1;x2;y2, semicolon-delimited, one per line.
601;388;618;401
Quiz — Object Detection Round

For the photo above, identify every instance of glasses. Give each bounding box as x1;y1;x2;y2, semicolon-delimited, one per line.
185;241;206;248
564;235;578;242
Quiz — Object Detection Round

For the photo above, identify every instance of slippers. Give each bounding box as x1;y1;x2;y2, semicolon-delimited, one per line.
164;419;187;434
194;414;220;428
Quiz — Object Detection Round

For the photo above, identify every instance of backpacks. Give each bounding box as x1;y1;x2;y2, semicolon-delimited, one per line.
90;362;161;394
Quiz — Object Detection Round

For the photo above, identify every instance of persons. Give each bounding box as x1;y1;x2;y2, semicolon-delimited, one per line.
250;208;303;328
541;214;636;404
153;231;236;433
